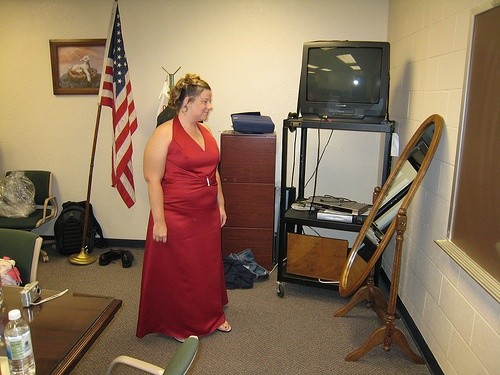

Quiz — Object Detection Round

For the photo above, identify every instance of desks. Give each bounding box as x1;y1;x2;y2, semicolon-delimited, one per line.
0;284;123;375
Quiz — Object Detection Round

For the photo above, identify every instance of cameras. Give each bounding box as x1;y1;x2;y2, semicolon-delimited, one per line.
20;281;41;307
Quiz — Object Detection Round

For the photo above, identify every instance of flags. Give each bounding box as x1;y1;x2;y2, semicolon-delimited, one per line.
97;0;139;209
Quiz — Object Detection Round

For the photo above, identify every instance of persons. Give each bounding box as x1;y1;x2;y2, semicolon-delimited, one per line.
135;73;234;344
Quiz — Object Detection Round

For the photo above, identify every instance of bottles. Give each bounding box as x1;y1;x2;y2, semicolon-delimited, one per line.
4;309;36;375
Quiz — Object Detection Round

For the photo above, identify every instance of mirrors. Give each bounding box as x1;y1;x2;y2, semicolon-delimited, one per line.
339;114;444;298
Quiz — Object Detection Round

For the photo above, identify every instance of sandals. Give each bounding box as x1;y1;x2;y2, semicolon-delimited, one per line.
216;320;231;332
174;336;188;343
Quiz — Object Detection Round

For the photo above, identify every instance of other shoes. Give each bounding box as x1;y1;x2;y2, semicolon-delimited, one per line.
121;250;134;268
99;249;123;266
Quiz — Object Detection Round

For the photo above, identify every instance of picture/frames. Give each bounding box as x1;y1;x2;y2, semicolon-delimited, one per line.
49;38;107;95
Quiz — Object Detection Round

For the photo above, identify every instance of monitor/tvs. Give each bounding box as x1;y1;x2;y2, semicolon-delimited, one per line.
299;41;391;125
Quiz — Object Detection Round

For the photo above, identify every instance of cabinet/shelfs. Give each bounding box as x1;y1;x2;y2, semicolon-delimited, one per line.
219;129;277;271
276;119;397;304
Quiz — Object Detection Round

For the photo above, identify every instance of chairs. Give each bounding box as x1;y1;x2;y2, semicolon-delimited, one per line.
0;228;43;287
105;335;200;375
0;169;58;262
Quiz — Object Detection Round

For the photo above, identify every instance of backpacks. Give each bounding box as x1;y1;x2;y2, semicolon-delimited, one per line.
54;201;103;255
